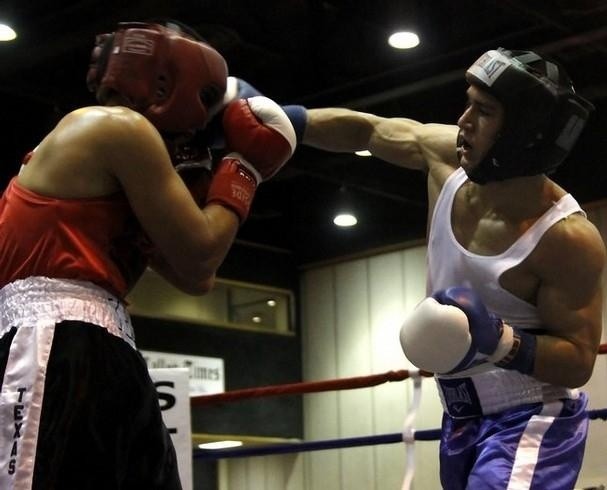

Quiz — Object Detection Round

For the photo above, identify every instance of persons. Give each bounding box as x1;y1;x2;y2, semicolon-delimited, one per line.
0;22;299;490
220;48;606;489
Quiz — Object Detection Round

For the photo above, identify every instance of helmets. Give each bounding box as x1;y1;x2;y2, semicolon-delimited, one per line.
87;25;229;134
457;48;595;186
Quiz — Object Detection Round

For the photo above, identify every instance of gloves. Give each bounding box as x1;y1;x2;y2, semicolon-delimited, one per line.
223;76;307;144
400;286;537;374
207;96;297;224
